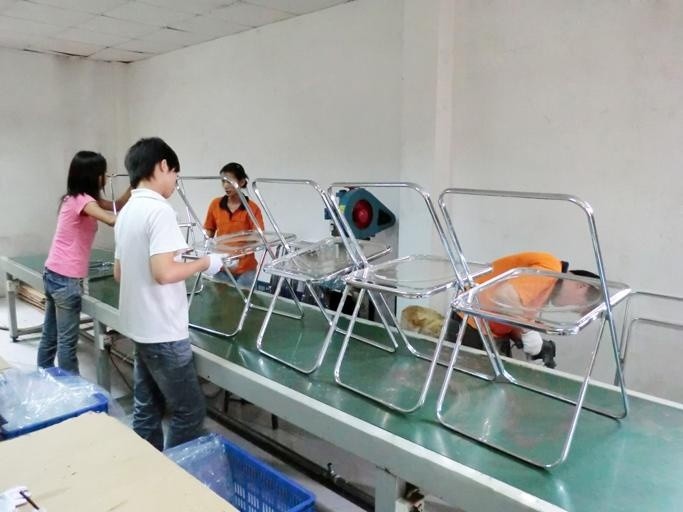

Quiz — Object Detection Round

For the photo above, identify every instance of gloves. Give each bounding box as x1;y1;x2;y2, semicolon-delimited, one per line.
521;330;543;356
203;253;222;276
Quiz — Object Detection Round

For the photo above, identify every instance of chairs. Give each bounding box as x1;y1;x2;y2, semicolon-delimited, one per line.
175;173;305;338
436;185;635;470
253;177;398;375
110;173;205;296
326;182;500;414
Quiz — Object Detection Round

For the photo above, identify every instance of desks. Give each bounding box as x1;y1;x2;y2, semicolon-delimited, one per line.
1;249;683;512
0;411;240;511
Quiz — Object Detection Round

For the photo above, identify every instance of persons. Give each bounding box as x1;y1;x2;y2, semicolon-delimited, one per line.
202;161;266;287
448;253;603;358
37;151;134;375
114;137;211;451
